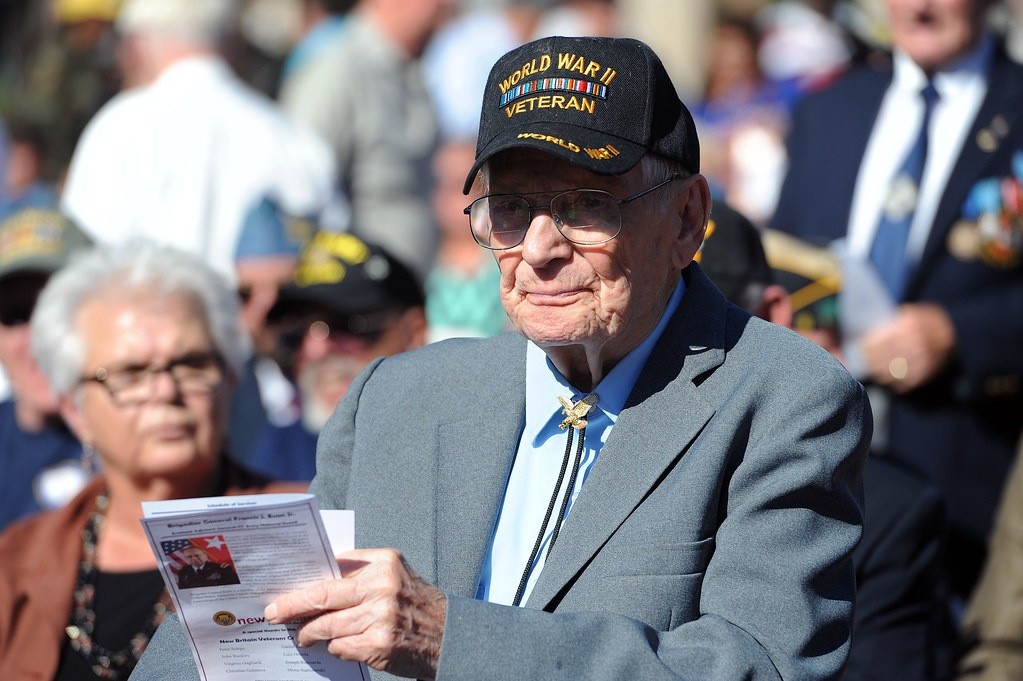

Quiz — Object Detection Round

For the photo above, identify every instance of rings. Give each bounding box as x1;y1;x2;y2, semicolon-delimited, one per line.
888;355;906;382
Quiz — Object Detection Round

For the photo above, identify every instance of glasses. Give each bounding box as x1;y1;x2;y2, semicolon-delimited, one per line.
75;347;228;401
462;172;684;247
276;324;385;356
227;280;256;315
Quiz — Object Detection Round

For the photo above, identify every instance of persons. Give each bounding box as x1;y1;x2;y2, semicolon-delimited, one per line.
0;239;356;681
132;35;873;681
0;0;853;523
741;0;1023;681
178;549;240;589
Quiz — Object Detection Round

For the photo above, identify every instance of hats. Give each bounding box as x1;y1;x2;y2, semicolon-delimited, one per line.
264;229;428;328
694;201;773;283
2;207;97;275
462;35;701;194
238;194;322;260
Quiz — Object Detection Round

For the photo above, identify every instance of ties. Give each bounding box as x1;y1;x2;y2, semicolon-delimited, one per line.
867;84;944;293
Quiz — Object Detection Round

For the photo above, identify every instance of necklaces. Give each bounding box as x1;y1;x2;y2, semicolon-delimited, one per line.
66;496;182;679
